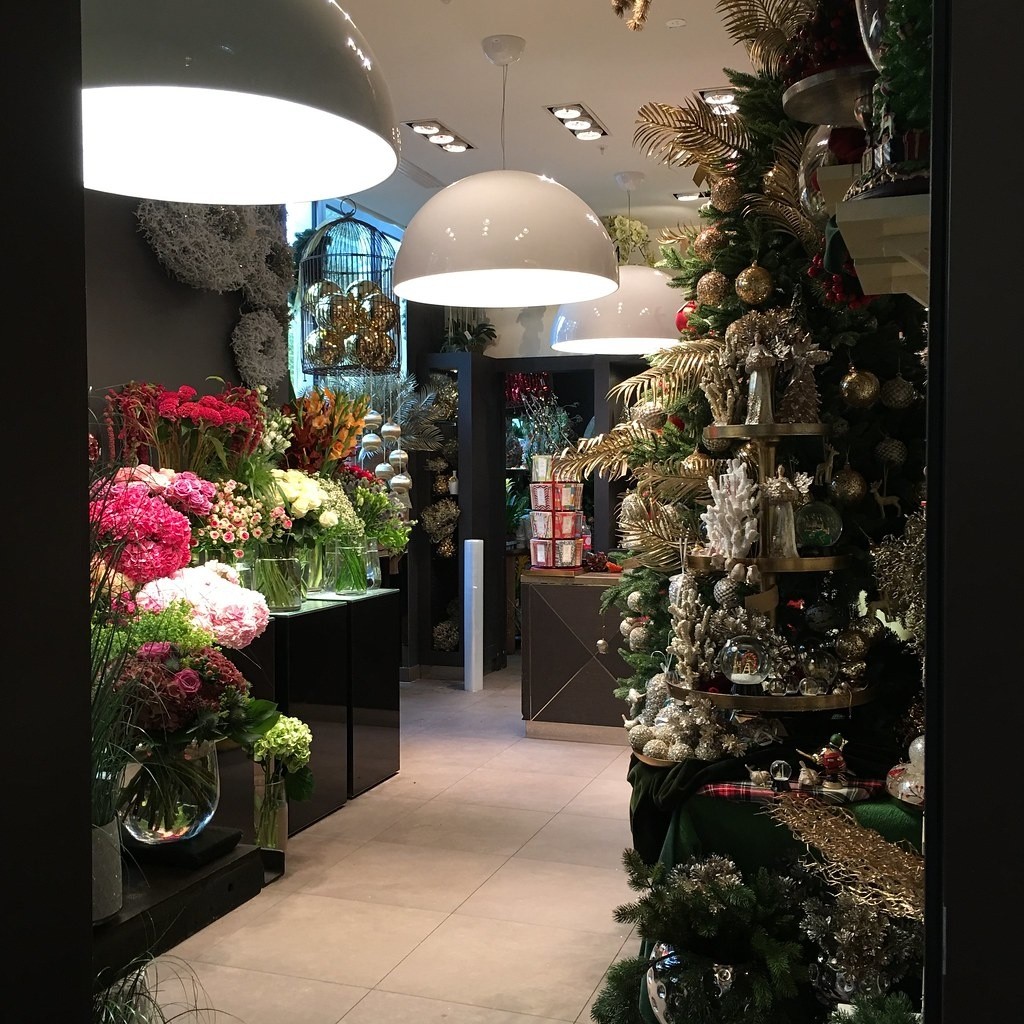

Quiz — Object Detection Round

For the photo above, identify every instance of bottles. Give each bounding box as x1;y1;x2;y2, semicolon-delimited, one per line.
580;514;592;560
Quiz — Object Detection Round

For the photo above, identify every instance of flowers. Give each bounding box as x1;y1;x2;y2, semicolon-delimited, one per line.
113;641;249;831
242;715;316;847
90;374;416;607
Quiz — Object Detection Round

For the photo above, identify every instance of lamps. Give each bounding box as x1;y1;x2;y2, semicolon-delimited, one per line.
82;0;399;205
388;32;621;308
547;172;701;359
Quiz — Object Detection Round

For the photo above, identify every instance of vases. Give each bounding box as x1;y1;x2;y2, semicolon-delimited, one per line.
227;534;383;613
92;818;122;920
243;775;288;851
115;740;221;847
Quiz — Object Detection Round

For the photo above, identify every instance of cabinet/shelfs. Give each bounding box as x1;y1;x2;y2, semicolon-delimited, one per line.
212;588;401;841
814;161;929;313
